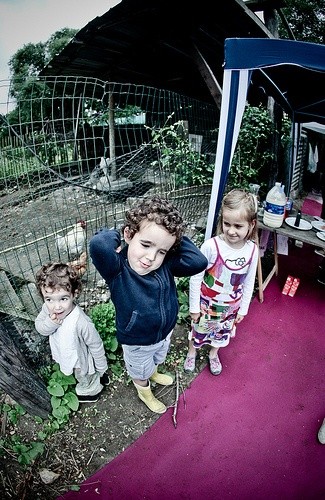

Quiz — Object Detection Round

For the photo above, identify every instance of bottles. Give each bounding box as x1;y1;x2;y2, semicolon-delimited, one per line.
262;182;287;228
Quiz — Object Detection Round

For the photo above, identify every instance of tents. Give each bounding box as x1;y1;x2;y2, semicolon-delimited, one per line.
206;36;325;240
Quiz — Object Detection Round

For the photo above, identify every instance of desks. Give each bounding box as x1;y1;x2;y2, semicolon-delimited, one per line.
254;212;325;304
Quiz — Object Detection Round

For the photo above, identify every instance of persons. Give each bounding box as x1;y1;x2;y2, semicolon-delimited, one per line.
185;190;260;375
89;195;209;415
34;260;111;403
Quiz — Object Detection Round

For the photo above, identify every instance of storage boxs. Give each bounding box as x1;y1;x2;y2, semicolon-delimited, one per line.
287;277;301;298
282;274;294;296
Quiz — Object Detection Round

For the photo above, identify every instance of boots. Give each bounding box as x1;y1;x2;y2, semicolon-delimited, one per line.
132;378;167;414
150;364;173;387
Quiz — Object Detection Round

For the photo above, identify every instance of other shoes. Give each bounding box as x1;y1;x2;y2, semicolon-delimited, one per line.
184;352;197;372
208;355;223;376
76;394;99;403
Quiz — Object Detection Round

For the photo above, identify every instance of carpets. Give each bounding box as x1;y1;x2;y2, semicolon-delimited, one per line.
57;244;325;500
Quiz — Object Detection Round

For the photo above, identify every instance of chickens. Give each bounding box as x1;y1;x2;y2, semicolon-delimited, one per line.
56;220;87;280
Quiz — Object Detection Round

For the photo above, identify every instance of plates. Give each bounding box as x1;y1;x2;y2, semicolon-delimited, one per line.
284;217;313;231
311;221;325;232
315;231;325;241
